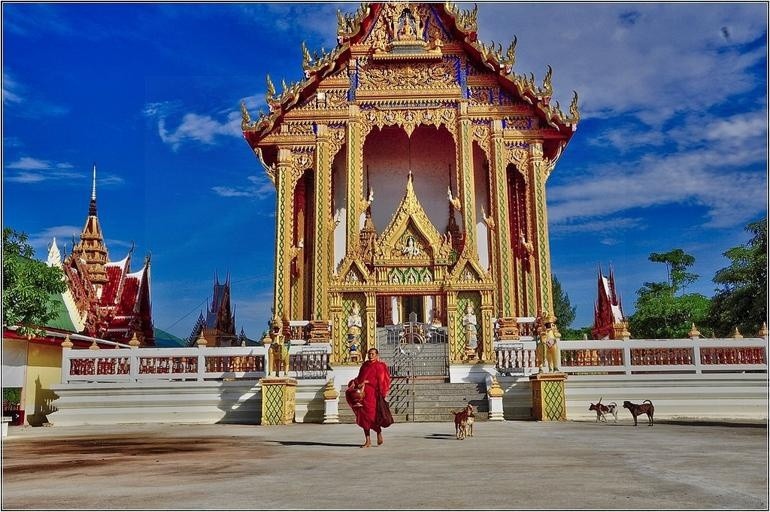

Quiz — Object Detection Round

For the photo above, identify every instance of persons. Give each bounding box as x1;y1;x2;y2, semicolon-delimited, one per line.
461;301;478;352
348;347;391;448
398;15;416;40
431;31;445;53
373;30;385;54
346;302;363;351
402;235;420;260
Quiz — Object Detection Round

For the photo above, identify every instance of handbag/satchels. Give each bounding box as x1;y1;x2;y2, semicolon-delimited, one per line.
376;390;394;428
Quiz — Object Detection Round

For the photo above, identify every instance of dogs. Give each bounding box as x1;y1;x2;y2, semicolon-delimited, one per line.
451;404;475;439
589;397;618;424
623;400;654;426
